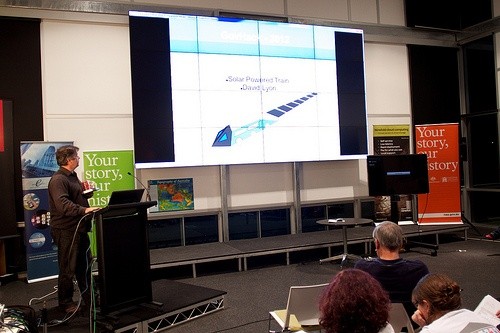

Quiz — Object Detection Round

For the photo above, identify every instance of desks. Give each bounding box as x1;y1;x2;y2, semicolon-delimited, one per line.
316;218;373;271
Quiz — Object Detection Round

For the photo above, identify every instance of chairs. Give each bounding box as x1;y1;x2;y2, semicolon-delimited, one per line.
268;282;329;333
387;303;415;333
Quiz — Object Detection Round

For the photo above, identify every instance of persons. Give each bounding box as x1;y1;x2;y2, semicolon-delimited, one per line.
353;221;430;333
412;271;500;333
496;310;500;318
48;145;103;313
316;269;395;333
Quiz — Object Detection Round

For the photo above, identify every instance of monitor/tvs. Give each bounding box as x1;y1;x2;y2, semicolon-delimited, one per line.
367;154;429;196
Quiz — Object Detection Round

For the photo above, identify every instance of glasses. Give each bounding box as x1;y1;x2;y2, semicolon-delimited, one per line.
68;156;80;162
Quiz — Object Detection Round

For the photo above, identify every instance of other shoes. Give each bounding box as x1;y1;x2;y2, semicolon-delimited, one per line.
65;304;82;314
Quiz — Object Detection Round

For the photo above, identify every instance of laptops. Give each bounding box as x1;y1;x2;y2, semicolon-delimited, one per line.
108;189;144;205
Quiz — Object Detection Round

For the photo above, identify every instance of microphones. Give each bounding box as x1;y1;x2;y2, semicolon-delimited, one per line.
128;172;151;201
